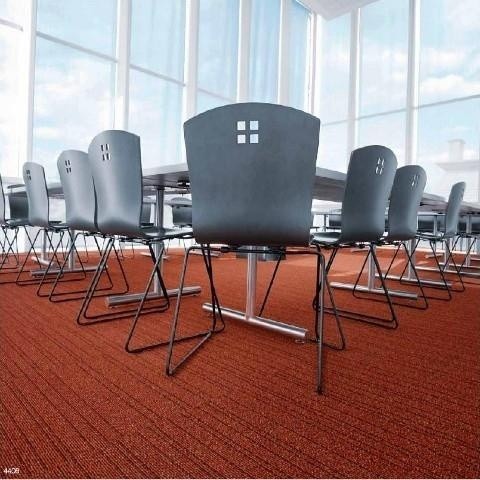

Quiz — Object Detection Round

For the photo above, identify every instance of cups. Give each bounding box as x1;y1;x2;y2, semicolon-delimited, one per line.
449;139;465;161
